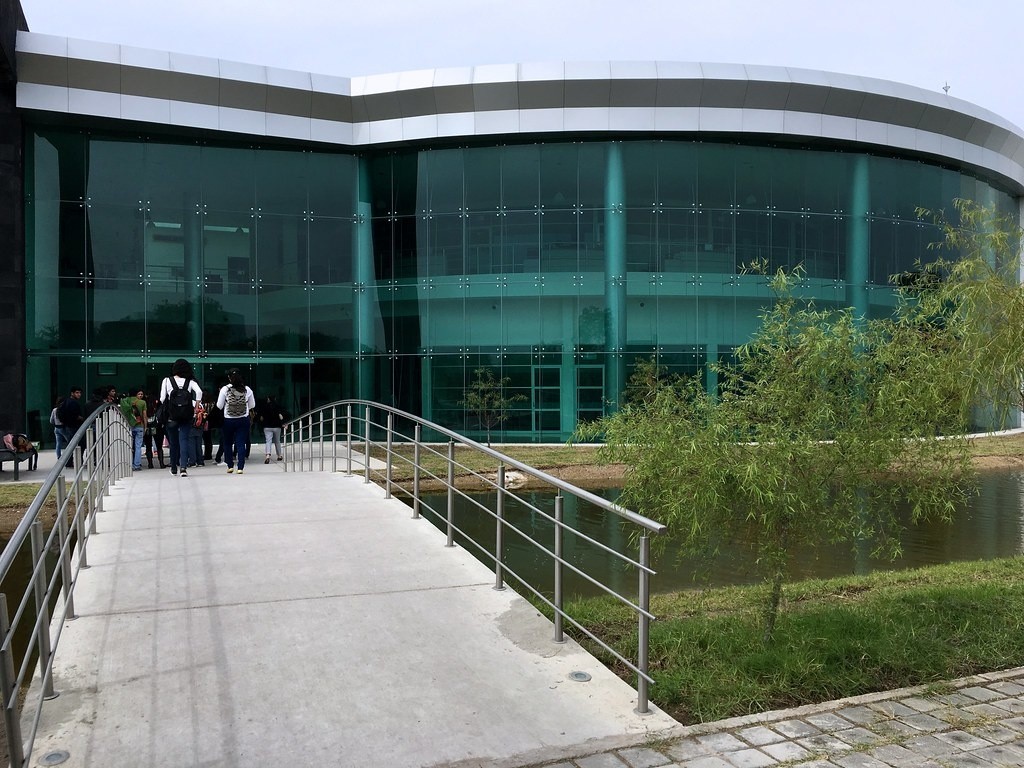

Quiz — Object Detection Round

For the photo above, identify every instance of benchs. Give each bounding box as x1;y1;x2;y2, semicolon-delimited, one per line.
0;431;34;480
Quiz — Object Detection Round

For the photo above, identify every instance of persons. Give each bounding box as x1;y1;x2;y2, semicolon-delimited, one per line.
259;394;285;464
46;383;167;469
162;380;255;468
216;367;256;474
160;359;203;477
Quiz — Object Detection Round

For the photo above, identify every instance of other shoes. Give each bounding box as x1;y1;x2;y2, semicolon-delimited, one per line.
180;468;186;476
278;456;282;460
169;466;177;475
135;465;142;471
227;468;233;473
198;463;203;466
187;463;196;467
65;464;74;467
237;469;243;474
265;455;270;464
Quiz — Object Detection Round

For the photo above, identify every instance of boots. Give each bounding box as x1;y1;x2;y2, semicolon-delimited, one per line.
146;451;154;468
158;450;166;469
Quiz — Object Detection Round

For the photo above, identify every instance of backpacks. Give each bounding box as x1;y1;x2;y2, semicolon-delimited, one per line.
3;433;37;453
132;398;142;424
224;385;247;416
168;375;194;425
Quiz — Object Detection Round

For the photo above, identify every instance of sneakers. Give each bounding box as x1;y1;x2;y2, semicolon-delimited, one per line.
211;460;218;463
217;461;228;466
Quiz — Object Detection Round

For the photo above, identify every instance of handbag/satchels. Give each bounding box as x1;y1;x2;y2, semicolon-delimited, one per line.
56;399;67;422
